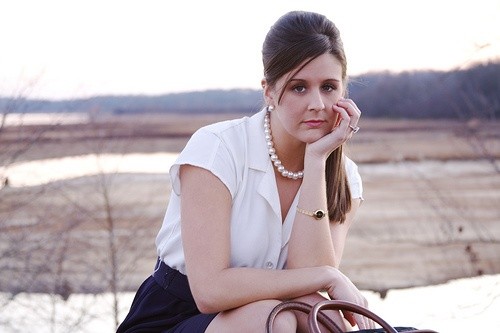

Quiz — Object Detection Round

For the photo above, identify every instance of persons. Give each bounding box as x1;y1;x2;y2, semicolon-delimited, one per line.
114;9;379;333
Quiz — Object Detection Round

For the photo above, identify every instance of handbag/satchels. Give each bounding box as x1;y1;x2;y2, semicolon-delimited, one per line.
266;300;439;333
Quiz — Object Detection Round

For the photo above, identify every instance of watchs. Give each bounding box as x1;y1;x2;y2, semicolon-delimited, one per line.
296;205;329;221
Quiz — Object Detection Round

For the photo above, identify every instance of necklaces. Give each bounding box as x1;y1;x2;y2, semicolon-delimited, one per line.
265;111;305;180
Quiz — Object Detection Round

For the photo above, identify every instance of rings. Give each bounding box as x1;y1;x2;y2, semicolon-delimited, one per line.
349;124;360;134
